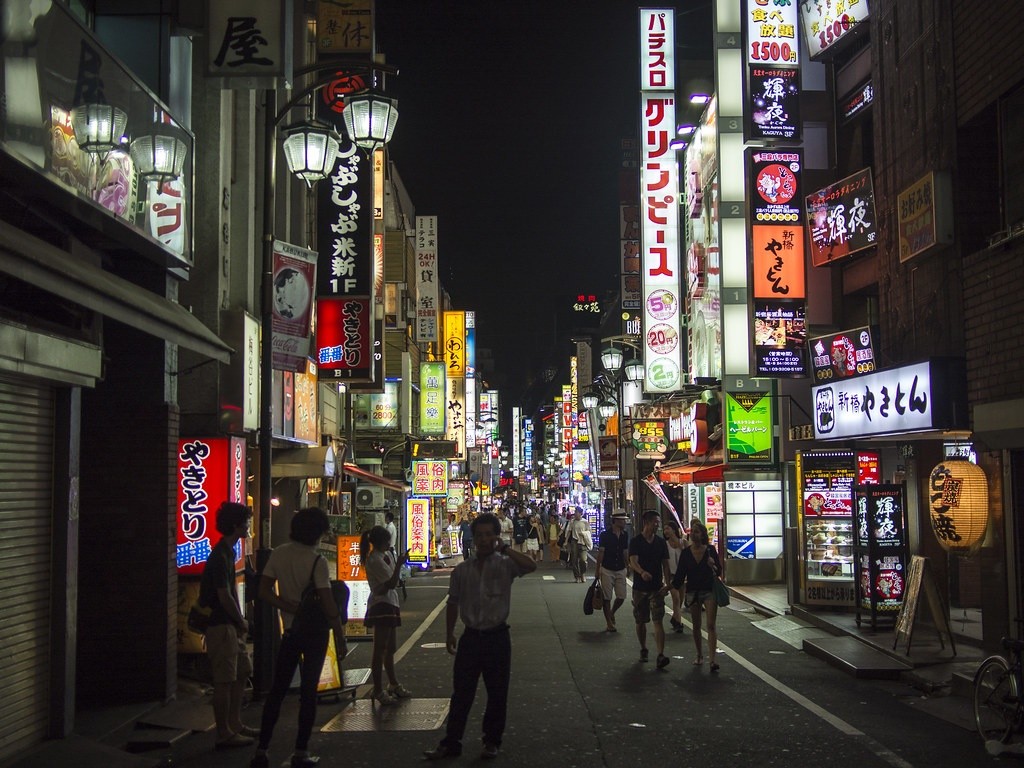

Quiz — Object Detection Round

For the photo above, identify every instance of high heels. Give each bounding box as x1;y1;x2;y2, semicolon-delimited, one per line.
387;683;413;698
371;690;398;706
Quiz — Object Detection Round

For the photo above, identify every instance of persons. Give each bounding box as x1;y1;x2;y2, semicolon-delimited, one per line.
385;495;595;584
359;526;414;706
594;509;633;633
198;500;263;746
627;512;672;668
421;513;538;761
252;506;347;764
663;521;724;672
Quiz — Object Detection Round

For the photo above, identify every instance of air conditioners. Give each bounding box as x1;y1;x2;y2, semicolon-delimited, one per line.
355;485;385;509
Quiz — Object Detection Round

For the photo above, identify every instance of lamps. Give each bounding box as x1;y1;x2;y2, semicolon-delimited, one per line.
652;377;721;406
689;93;711;105
707;424;722;441
669;139;689;151
676;121;697;135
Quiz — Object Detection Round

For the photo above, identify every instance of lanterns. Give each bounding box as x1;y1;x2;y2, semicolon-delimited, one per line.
929;456;989;554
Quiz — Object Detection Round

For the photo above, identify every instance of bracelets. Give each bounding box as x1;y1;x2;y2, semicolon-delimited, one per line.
626;564;630;568
500;544;511;555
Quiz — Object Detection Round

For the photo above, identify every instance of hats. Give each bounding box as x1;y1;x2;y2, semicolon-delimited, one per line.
609;508;629;519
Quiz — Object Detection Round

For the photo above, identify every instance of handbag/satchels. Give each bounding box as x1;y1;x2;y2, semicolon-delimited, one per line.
557;532;566;547
592;579;604;610
712;576;731;608
291;554;351;638
559;550;571;563
187;602;213;634
583;577;597;615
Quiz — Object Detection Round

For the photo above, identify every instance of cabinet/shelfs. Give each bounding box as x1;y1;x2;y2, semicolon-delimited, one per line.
805;515;856;582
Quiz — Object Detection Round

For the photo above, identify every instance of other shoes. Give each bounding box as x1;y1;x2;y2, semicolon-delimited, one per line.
609;610;616;625
607;626;617;632
710;661;720;672
214;732;256;748
692;654;703;665
481;744;499;758
656;653;670;668
290;754;320;768
239;725;262;737
574;578;580;583
250;749;270;768
581;574;586;583
639;648;649;662
670;616;684;632
423;737;464;760
